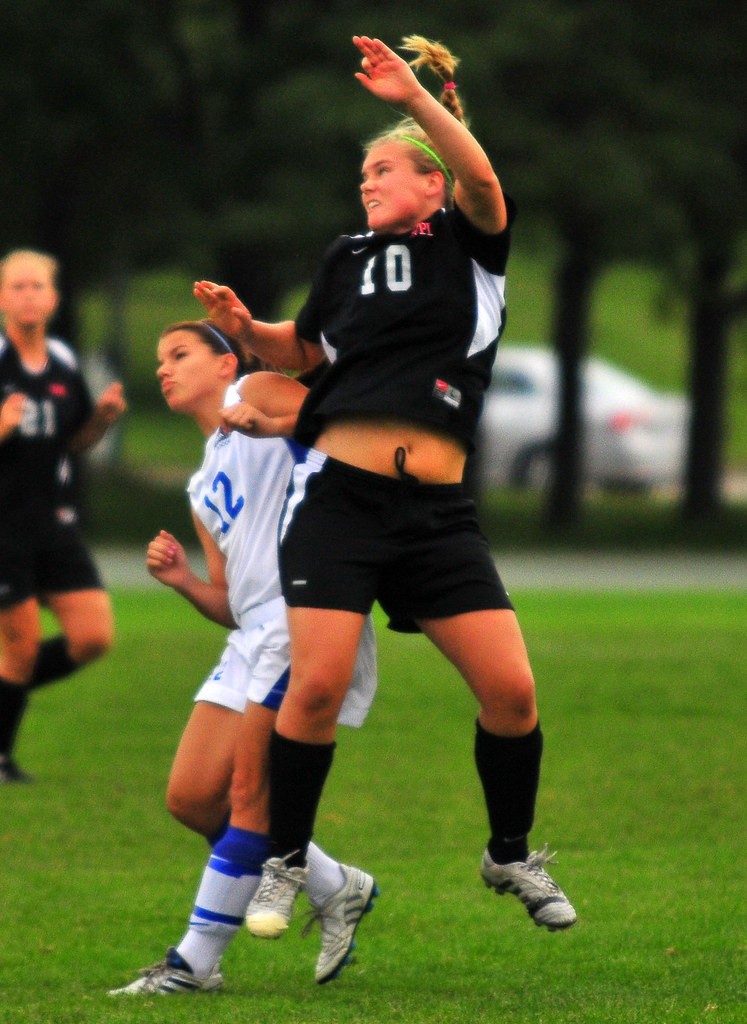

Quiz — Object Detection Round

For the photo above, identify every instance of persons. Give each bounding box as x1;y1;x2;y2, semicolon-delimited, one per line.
109;319;379;996
0;251;127;784
195;34;576;939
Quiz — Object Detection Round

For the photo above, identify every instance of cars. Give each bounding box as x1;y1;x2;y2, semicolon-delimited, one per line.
477;344;690;497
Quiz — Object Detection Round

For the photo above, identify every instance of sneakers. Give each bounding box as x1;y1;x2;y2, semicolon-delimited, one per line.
105;946;224;997
479;843;577;931
302;863;381;984
245;854;309;940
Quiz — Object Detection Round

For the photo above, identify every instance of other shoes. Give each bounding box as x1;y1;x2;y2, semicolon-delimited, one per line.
0;759;28;784
